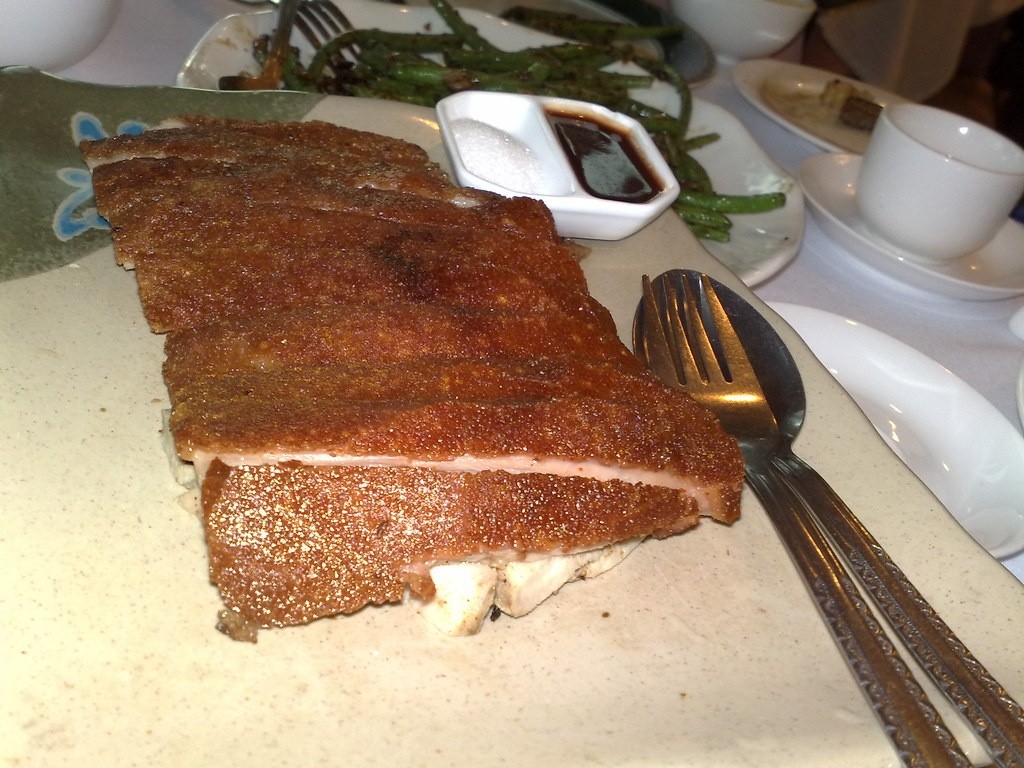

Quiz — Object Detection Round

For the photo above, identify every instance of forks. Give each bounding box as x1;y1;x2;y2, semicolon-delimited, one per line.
236;0;368;73
631;270;979;767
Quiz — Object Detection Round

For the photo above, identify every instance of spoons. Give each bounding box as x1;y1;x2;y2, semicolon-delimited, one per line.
630;269;1023;767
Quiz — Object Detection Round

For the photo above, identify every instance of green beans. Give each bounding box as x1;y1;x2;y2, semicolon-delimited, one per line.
250;0;785;243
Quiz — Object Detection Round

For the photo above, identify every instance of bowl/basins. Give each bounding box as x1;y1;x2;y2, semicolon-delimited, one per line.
670;0;819;62
0;0;119;72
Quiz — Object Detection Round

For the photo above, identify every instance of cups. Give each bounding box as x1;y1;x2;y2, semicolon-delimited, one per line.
852;98;1024;268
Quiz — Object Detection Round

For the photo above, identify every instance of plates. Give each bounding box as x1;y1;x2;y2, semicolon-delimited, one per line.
595;0;718;85
729;57;926;156
1;62;1022;765
791;149;1024;303
401;0;665;71
168;3;803;296
757;298;1024;568
